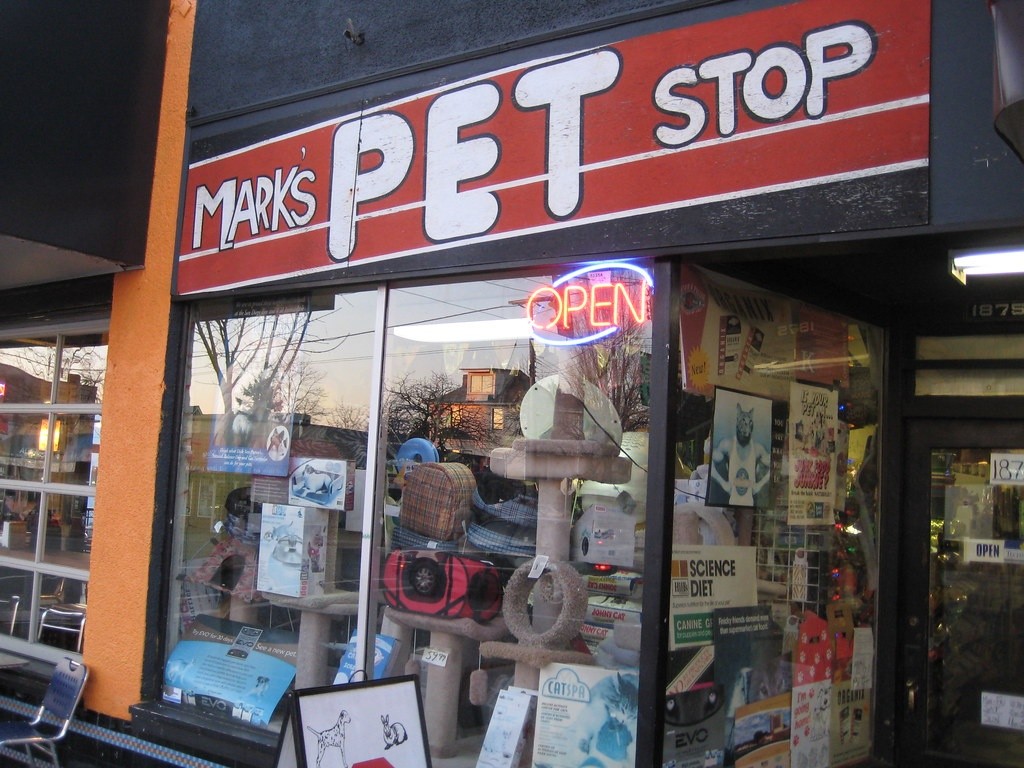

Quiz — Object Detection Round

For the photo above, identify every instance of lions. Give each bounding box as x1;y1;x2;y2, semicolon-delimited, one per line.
212;338;289;451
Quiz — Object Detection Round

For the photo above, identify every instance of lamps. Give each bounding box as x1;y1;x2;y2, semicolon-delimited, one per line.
947;244;1024;285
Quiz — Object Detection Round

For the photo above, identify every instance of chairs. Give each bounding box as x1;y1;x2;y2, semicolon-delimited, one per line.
0;657;90;768
0;570;87;653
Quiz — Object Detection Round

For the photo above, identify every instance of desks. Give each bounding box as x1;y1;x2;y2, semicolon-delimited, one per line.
0;652;30;670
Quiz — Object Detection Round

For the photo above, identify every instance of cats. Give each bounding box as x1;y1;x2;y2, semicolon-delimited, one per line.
578;672;639;754
735;403;755;444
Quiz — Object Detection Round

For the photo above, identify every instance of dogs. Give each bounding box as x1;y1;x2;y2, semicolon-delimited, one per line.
265;429;287;456
300;464;332;497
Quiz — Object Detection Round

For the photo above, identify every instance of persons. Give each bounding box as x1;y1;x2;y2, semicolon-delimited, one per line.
710;403;770;505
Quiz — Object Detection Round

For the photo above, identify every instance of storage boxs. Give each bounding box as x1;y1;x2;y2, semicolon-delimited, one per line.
569;504;635;567
257;503;329;597
289;456;357;512
334;629;402;685
475;685;539;768
532;661;640;768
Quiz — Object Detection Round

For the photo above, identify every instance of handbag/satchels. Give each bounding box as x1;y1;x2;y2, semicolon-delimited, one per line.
398;462;479;541
380;548;503;623
